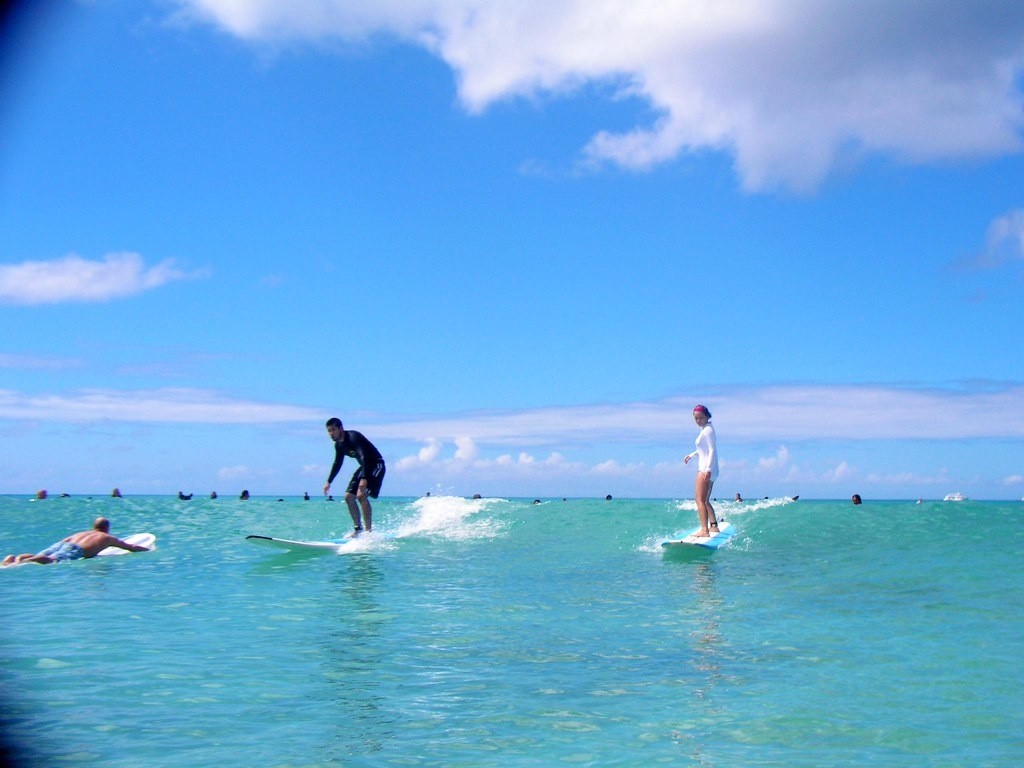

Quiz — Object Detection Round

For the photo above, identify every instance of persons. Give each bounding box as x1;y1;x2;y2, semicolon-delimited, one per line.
304;492;310;501
112;489;122;498
37;489;47;499
606;495;612;500
735;494;742;502
324;417;385;540
240;490;249;500
211;492;217;499
684;405;719;537
852;495;861;504
2;517;150;564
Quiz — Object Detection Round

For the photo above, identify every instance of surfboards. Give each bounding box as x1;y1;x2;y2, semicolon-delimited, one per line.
96;532;156;556
660;520;736;551
245;534;353;548
